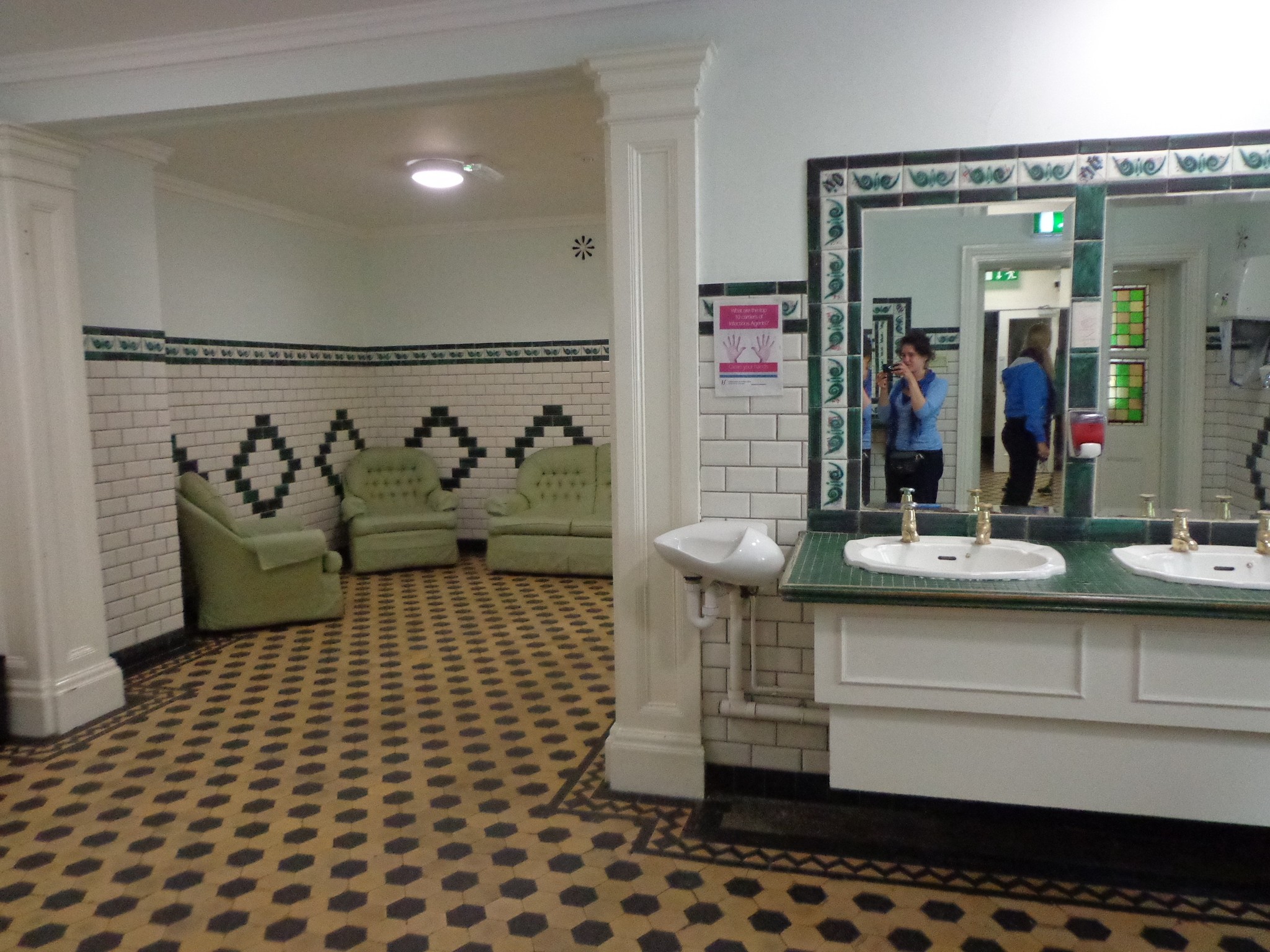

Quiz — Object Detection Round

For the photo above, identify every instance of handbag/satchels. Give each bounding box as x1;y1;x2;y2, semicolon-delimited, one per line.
889;450;928;476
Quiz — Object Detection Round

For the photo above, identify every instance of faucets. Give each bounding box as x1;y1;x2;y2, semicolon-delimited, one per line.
898;487;916;506
899;502;921;543
1140;493;1158;518
1216;494;1234;519
973;503;993;546
1256;508;1270;555
1168;507;1199;552
967;488;980;511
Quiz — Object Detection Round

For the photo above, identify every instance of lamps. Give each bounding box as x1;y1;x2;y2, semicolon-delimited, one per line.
409;159;464;189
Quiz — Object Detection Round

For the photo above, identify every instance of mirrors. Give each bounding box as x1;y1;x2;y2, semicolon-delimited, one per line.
860;199;1078;519
1092;189;1269;522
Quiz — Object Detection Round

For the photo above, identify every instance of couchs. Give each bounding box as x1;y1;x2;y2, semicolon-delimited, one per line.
175;471;343;629
486;441;612;576
341;446;461;572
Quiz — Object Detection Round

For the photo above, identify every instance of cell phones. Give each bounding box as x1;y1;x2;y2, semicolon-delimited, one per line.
883;363;899;372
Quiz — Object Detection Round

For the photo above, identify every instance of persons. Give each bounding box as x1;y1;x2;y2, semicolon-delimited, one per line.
861;331;949;505
1000;322;1057;506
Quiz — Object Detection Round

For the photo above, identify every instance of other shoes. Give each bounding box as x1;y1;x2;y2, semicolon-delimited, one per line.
1038;486;1053;496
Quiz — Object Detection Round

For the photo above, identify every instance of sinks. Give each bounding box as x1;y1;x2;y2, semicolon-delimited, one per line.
843;534;1066;580
654;517;786;587
1109;540;1270;592
